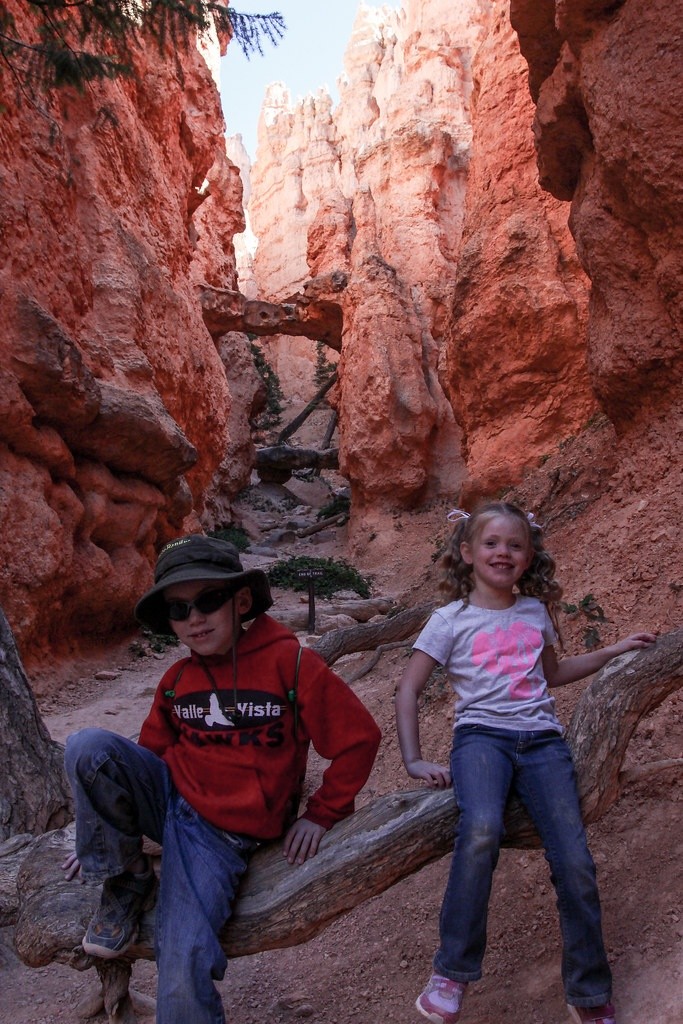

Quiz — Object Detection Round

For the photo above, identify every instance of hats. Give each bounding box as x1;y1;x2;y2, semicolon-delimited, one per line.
134;535;274;635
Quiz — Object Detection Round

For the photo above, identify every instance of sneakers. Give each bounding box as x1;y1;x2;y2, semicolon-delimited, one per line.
82;853;160;959
567;998;616;1024
415;970;467;1024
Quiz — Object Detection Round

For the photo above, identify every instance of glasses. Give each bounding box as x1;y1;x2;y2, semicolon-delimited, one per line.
162;585;237;622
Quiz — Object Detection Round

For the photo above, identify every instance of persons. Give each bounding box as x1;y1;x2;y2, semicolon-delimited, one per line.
395;501;658;1024
61;533;383;1024
